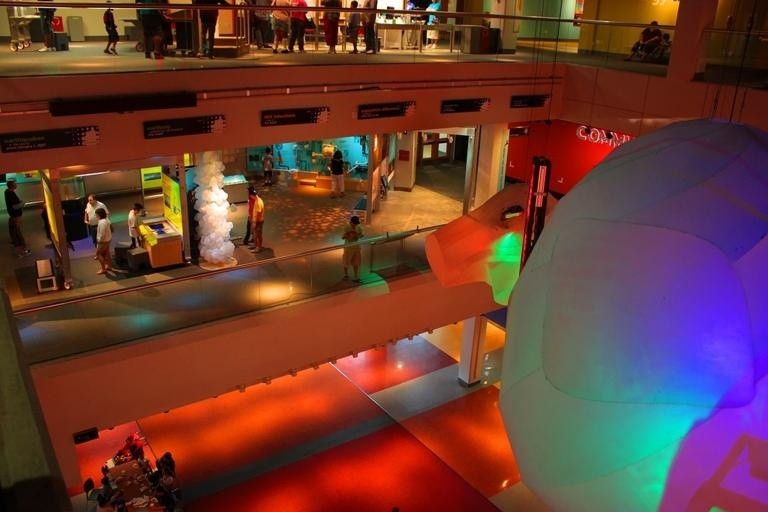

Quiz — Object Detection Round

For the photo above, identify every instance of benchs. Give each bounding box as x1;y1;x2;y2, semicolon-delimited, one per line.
35;258;58;293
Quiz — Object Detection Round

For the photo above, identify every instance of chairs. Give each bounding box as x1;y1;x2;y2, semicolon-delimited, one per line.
83;443;179;511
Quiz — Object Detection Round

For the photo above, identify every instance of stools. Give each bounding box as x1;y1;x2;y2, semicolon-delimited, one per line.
127;246;151;273
114;240;134;264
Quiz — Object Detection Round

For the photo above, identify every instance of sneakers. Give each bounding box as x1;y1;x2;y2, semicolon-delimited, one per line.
425;43;440;50
145;51;217;59
352;278;363;283
271;48;307;55
96;265;112;275
240;237;264;254
342;274;349;281
327;47;377;55
103;48;119;57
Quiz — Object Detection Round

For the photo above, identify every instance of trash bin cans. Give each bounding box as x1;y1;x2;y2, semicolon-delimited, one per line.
68;15;85;42
456;25;500;54
29;15;64;42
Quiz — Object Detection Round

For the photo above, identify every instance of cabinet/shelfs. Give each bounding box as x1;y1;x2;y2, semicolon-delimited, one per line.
136;219;183;267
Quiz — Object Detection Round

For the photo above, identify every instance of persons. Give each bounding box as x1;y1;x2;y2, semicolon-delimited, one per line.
623;20;661;62
325;149;347;198
127;203;148;249
237;186;257;245
246;190;264;253
133;0;166;62
247;1;442;56
156;0;177;58
33;0;57;54
94;207;112;275
4;179;25;246
102;0;120;55
8;217;30;261
261;147;274;187
84;193;110;260
115;435;181;512
654;33;672;63
340;216;363;284
40;201;54;249
190;0;231;60
